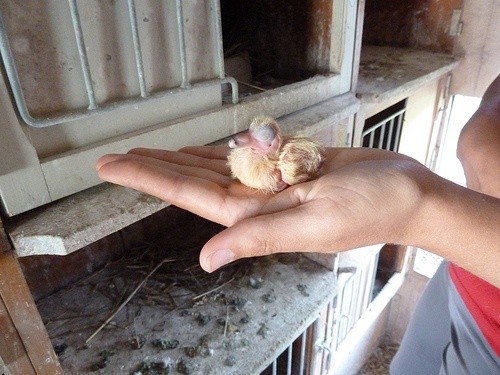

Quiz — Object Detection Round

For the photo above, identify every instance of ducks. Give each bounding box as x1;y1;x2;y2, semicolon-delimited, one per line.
224;115;327;198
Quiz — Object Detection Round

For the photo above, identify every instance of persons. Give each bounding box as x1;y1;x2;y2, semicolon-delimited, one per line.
96;74;500;375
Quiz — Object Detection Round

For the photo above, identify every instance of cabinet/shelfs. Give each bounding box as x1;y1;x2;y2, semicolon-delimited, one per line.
0;0;463;374
